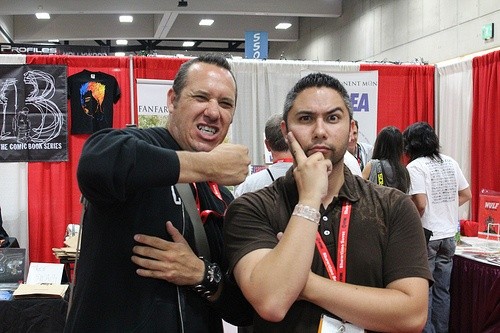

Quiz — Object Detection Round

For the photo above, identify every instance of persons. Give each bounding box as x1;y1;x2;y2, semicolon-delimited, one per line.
63;56;250;333
403;121;471;333
362;125;410;193
224;72;436;333
234;113;293;199
346;118;374;172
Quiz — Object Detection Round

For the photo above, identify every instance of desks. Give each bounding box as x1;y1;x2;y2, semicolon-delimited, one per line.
0;281;71;333
450;236;500;333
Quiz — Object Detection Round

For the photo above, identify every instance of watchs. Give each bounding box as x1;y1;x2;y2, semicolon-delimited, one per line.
193;256;224;299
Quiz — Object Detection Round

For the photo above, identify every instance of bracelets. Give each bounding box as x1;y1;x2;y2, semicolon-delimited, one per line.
292;205;321;224
